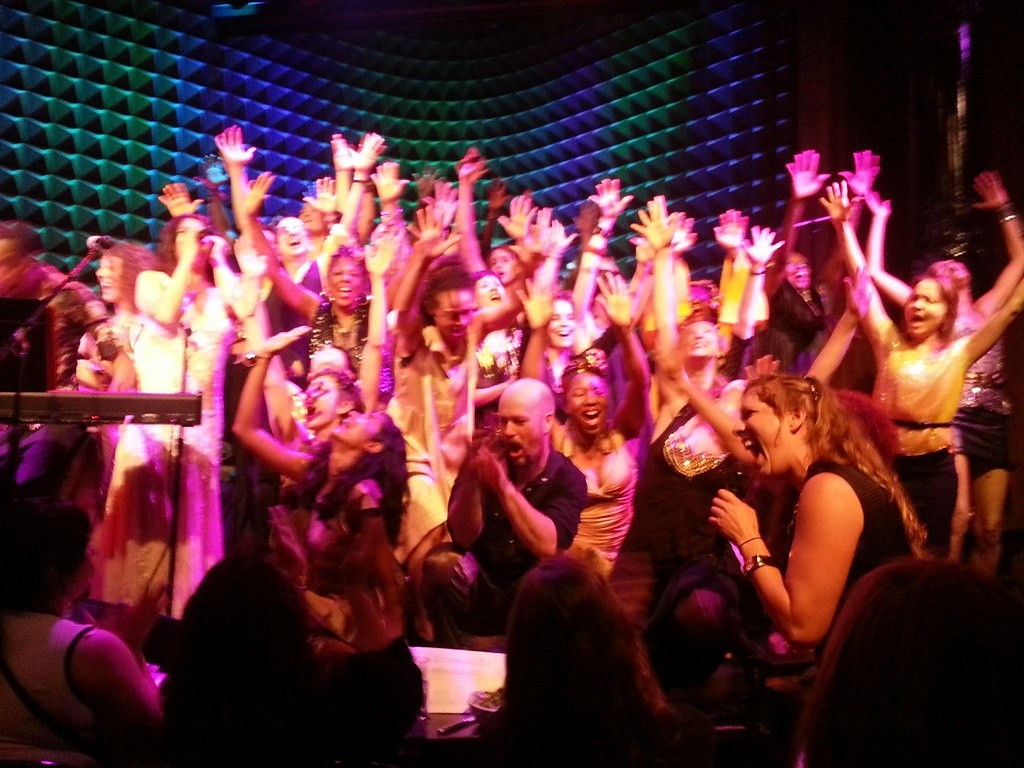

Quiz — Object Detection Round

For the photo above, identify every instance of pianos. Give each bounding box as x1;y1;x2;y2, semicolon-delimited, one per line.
0;390;204;427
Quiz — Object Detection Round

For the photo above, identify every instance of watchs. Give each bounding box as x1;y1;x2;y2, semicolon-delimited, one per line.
742;554;781;581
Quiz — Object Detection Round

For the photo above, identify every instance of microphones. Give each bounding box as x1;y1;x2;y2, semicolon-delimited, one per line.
87;236;115;251
710;471;749;559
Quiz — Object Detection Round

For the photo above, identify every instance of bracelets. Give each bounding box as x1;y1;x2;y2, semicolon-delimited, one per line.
350;178;373;191
738;536;763;547
999;213;1017;225
592;225;610;240
580;247;608;257
750;270;766;275
233;349;272;370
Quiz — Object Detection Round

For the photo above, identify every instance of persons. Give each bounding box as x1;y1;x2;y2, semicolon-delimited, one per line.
0;118;1024;768
